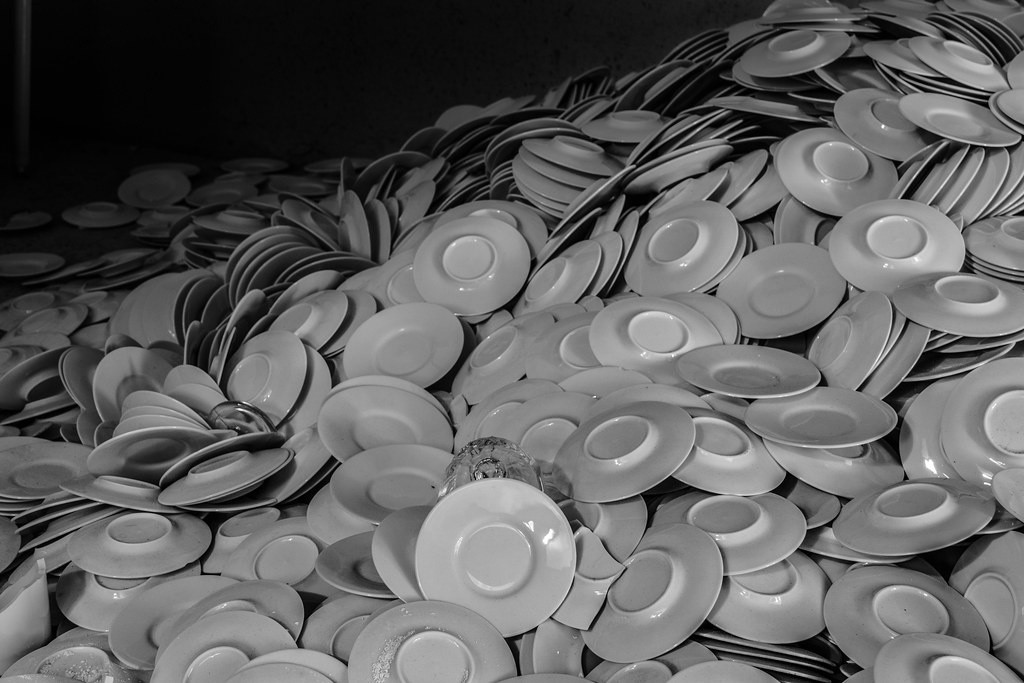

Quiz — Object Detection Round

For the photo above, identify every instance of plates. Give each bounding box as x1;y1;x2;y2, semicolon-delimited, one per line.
0;0;1024;683
